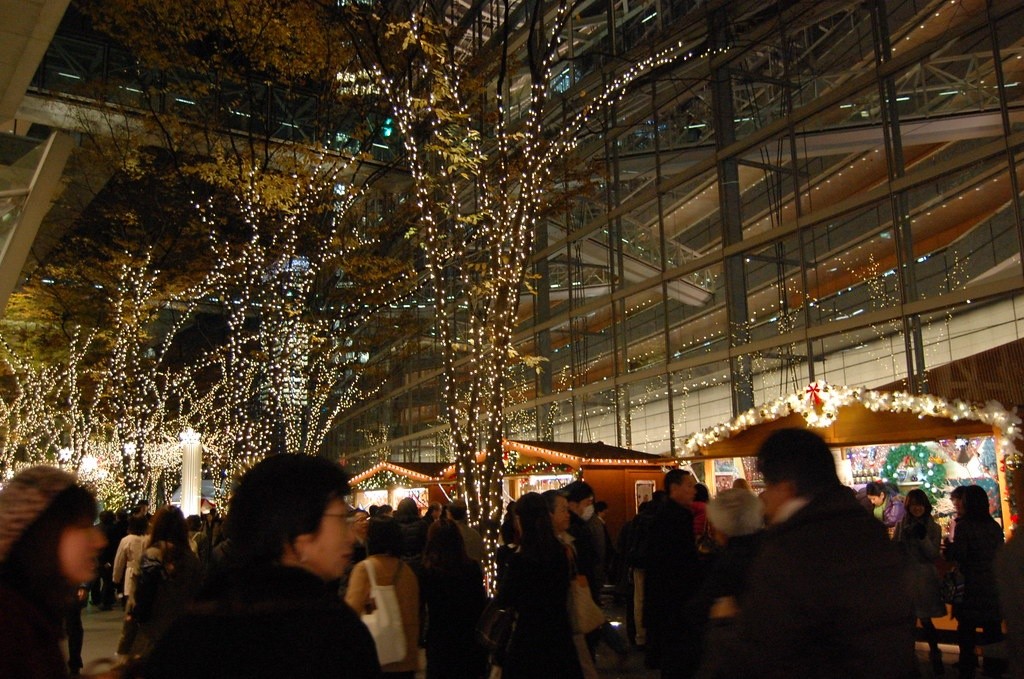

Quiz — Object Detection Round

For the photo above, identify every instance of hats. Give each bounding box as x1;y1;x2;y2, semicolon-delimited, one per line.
0;467;77;565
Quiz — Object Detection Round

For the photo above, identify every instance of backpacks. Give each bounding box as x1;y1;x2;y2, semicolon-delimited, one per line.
188;530;202;560
396;520;424;567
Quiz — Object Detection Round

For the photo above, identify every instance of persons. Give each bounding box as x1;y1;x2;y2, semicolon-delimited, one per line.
139;452;382;679
86;469;933;677
0;466;106;677
715;430;920;677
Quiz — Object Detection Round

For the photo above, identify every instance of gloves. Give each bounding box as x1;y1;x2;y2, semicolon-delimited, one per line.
913;520;927;540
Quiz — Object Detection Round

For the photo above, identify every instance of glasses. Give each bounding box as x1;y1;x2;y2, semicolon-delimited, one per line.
356;516;372;523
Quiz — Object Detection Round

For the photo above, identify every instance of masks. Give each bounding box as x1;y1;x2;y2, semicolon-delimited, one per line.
574;504;595;522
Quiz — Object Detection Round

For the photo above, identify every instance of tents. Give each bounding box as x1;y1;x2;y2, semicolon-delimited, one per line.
698;382;1021;558
441;440;679;547
347;460;453;520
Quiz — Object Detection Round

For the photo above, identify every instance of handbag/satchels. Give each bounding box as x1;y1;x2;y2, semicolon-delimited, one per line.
359;559;407;666
563;540;604;633
474;602;517;652
938;568;966;606
